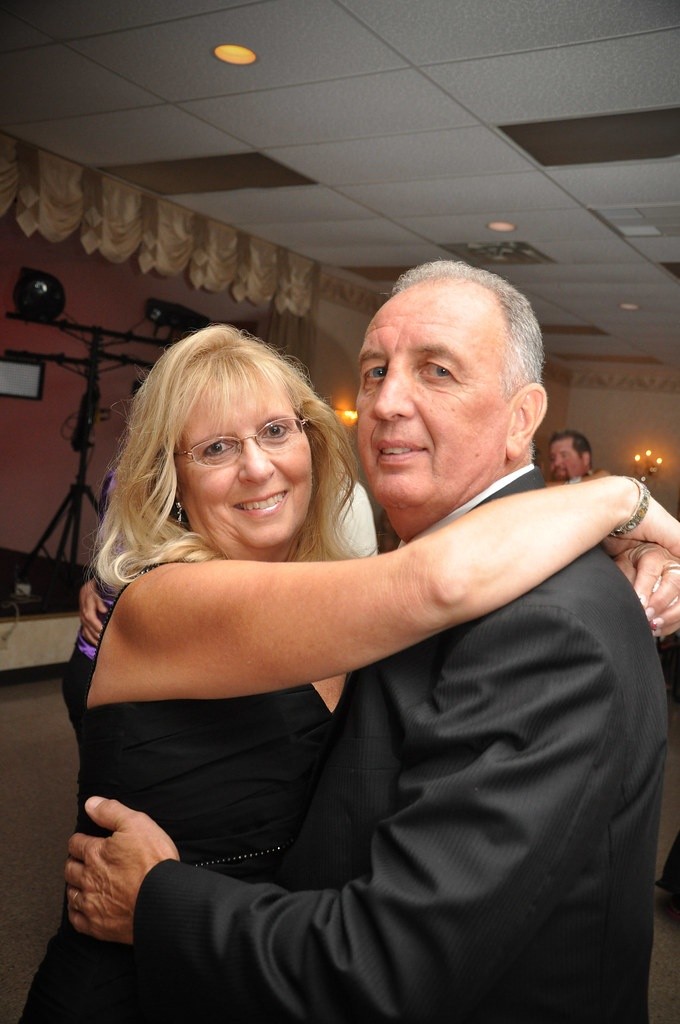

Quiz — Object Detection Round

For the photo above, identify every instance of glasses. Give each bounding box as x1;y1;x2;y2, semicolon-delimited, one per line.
173;417;309;469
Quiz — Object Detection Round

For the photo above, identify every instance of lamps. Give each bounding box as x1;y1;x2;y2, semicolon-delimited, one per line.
633;450;663;485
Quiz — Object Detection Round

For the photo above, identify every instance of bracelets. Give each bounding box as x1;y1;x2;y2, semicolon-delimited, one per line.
609;477;650;537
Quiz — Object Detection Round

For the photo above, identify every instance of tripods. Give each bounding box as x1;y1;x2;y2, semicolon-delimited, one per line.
13;327;133;612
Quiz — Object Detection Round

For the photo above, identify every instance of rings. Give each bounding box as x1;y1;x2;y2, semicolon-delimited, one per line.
73;892;80;912
661;565;680;575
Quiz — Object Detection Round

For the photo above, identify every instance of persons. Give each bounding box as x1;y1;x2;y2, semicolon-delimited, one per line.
64;260;669;1024
656;831;679;920
535;449;610;486
549;431;593;480
17;324;680;1024
